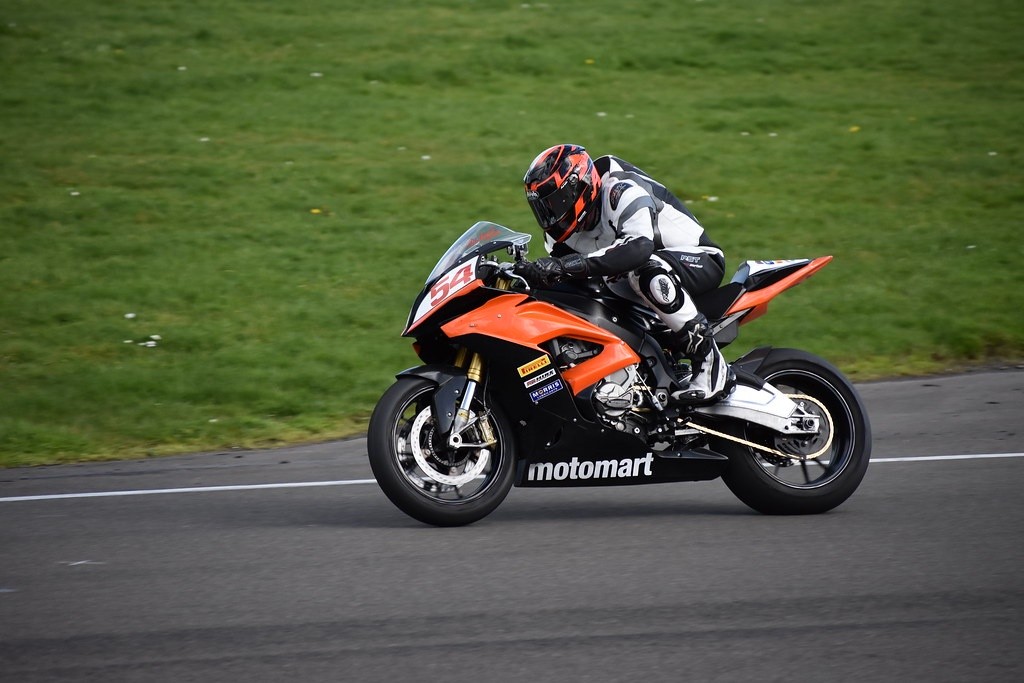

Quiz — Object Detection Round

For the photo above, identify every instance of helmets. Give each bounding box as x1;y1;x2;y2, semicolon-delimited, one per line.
522;144;602;244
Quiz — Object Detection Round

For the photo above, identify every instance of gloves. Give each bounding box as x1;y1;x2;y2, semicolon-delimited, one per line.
521;253;589;291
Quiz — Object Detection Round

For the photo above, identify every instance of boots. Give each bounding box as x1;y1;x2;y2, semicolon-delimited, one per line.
670;310;737;408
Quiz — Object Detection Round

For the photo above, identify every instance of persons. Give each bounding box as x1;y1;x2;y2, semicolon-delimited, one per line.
523;144;736;405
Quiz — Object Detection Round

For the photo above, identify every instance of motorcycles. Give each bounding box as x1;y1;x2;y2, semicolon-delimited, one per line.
366;220;874;529
